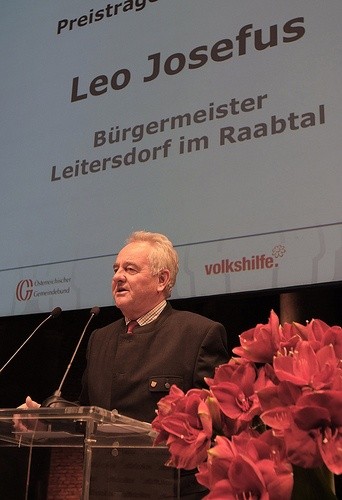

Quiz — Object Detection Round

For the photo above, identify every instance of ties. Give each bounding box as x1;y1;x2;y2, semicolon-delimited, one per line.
127;321;138;334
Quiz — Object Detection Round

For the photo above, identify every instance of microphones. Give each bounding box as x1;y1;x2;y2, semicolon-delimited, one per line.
41;305;100;407
0;306;62;372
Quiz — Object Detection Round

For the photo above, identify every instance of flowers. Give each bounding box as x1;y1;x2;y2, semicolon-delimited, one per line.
148;309;342;500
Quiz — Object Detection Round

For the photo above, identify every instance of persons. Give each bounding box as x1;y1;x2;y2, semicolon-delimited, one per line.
13;231;230;500
